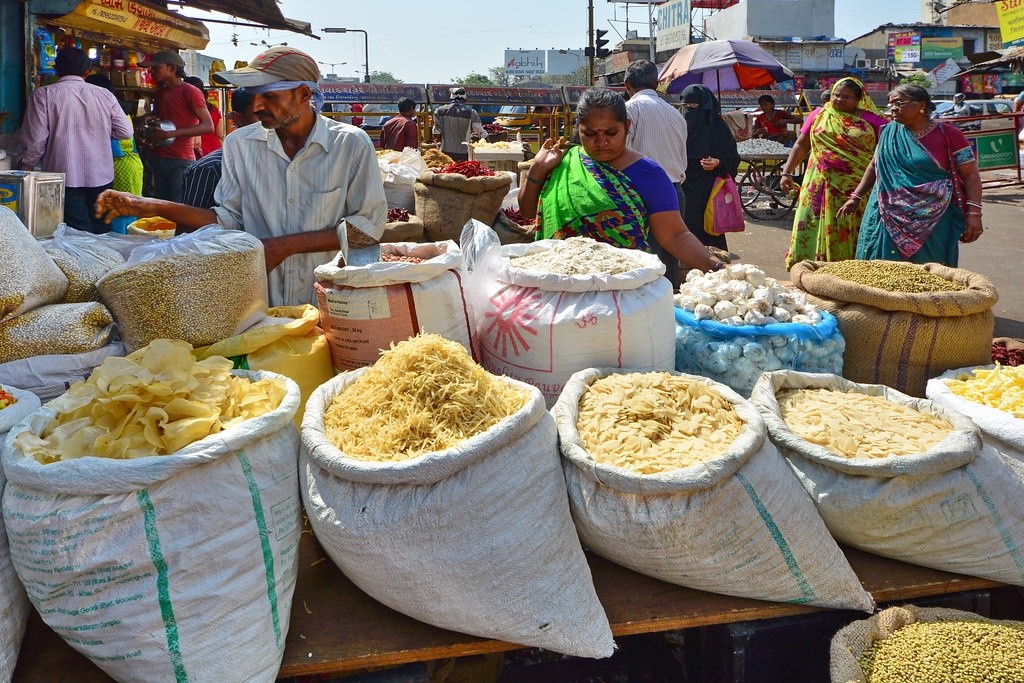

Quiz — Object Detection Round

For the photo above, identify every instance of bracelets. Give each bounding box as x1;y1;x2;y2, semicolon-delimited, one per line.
712;261;725;272
525;174;546;184
966;200;983;216
849;192;863;201
781;173;792;176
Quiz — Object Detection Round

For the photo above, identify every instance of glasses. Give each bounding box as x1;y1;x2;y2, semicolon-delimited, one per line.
887;99;919;109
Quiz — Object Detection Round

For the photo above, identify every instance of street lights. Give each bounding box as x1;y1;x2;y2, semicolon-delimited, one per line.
318;61;346;74
559;50;581;86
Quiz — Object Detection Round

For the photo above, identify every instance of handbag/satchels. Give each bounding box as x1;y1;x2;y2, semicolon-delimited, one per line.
704;173;745;236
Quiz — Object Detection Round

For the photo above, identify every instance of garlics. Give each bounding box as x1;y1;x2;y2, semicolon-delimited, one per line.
737;137;792;155
672;263;845;395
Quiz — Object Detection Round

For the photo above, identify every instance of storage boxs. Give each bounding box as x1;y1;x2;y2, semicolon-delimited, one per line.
1;170;67;240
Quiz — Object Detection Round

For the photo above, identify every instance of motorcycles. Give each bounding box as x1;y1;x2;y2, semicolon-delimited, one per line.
492;105;554;132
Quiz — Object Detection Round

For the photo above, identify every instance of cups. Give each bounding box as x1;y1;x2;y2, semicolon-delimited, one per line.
113;59;125;69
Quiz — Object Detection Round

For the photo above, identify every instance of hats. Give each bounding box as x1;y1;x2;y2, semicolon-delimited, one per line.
211;46;320;87
136;51;185;68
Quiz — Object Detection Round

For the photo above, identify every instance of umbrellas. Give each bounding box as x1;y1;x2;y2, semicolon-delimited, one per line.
656;39;795;115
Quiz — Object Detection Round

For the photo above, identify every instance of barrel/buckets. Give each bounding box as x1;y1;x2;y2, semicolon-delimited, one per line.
146;119;176;146
112;216;137;235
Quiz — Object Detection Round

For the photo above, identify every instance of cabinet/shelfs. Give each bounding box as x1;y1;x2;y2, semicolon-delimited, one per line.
110;86;154;147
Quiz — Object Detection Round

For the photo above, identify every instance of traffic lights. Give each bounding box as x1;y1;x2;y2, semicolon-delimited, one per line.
596;30;609;58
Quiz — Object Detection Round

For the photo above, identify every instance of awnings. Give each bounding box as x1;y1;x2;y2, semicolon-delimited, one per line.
165;0;320;41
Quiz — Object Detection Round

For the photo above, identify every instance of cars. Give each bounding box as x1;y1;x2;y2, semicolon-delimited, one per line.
880;94;1024;170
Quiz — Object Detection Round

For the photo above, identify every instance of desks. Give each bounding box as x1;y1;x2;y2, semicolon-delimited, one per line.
10;511;1009;683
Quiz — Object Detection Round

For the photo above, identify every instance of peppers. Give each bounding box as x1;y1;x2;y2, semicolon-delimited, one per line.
388;207;410;222
432;159;495;177
991;343;1024;367
482;122;504;135
501;205;535;226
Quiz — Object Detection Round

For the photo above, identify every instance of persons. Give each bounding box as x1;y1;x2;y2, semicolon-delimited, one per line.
1014;90;1024;127
518;87;730;273
623;59;687;281
936;92;970;124
836;85;984;267
433;87;488;162
679;86;740;251
780;77;889;271
380;98;417;152
754;94;803;143
16;44;260;237
95;46;388;308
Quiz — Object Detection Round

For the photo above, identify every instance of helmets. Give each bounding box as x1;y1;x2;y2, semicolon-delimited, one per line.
952;93;966;104
